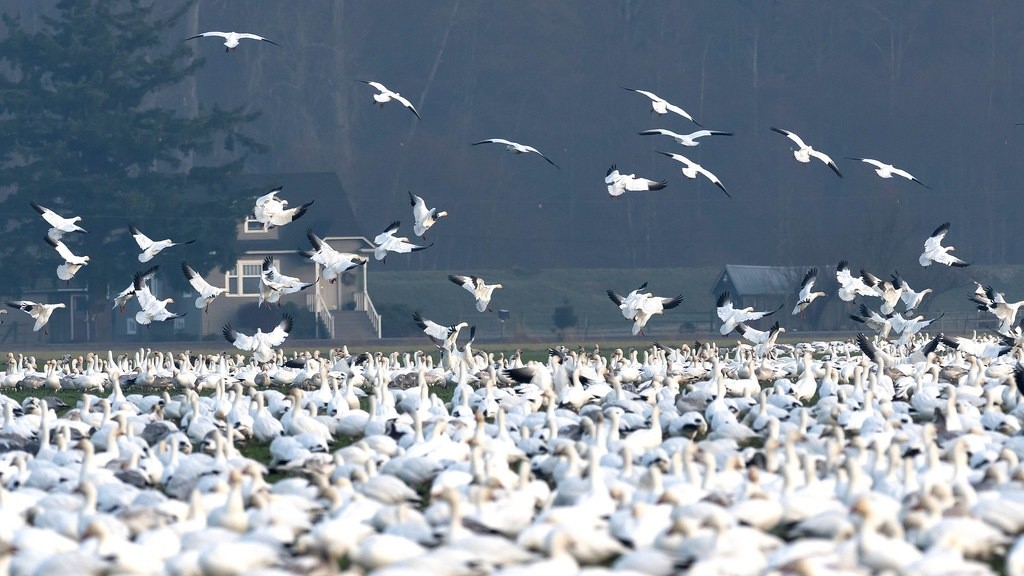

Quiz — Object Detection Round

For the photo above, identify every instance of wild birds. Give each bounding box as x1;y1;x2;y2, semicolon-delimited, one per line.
411;311;469;351
845;304;891;340
219;312;294;363
406;190;449;240
447;273;504;314
28;200;92;239
184;30;283;54
606;281;685;336
620;86;703;127
888;311;945;336
771;127;843;179
967;279;1024;333
356;78;421;121
251;185;315;230
298;227;368;283
373;220;434;265
603;163;669;201
471;135;560;170
792;267;829;318
134;269;188;325
715;291;784;336
43;236;92;281
636;128;736;147
258;255;320;310
111;265;160;312
656;150;733;200
834;262;933;317
844;157;924;187
128;224;198;262
181;260;232;314
0;320;1024;576
2;298;67;335
919;222;972;269
